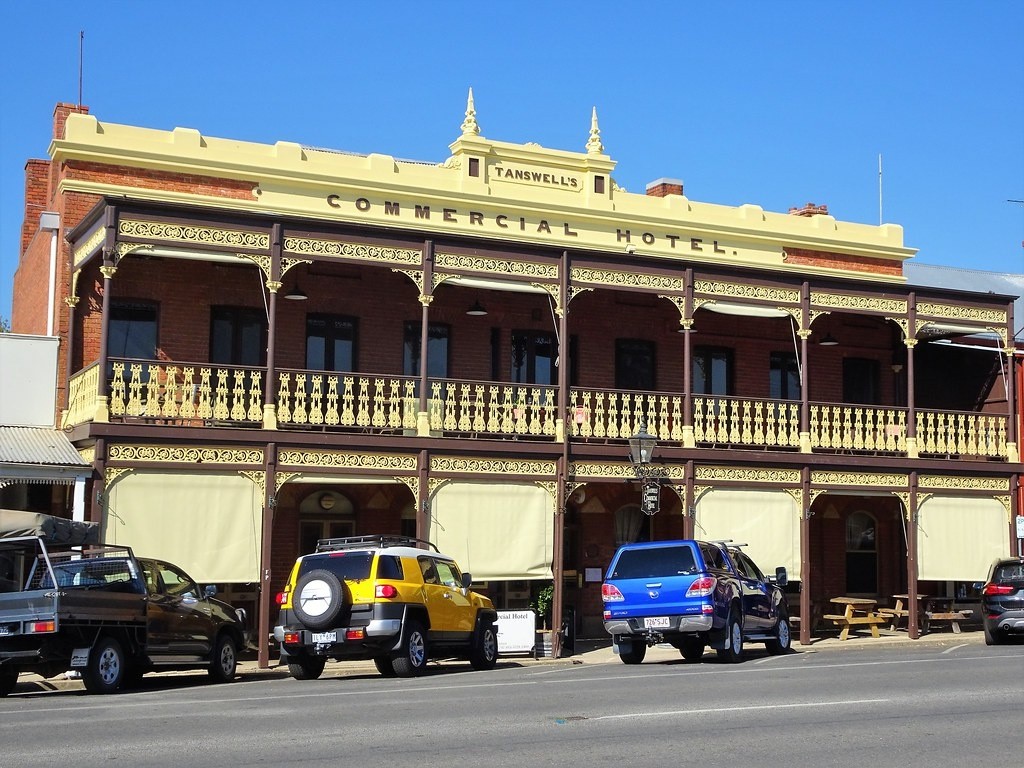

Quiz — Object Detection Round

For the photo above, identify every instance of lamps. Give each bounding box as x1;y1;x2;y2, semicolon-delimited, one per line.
284;264;309;300
466;288;490;317
622;416;658;484
319;493;337;511
678;322;698;335
574;486;586;504
819;315;840;346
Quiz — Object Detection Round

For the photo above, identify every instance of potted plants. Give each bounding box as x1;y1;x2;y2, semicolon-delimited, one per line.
527;580;567;660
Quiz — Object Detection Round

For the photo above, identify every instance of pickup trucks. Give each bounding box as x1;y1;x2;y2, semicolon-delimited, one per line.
0;535;249;699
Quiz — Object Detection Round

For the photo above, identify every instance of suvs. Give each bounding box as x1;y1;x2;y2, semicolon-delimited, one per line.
274;534;499;681
600;538;792;665
973;556;1024;646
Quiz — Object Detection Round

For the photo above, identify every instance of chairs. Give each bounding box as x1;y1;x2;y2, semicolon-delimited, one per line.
144;570;167;595
303;554;403;579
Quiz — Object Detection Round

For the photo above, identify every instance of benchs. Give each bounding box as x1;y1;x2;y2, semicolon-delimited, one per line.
789;606;975;628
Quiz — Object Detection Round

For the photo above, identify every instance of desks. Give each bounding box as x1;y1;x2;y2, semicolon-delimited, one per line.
810;596;882;640
890;593;930;634
